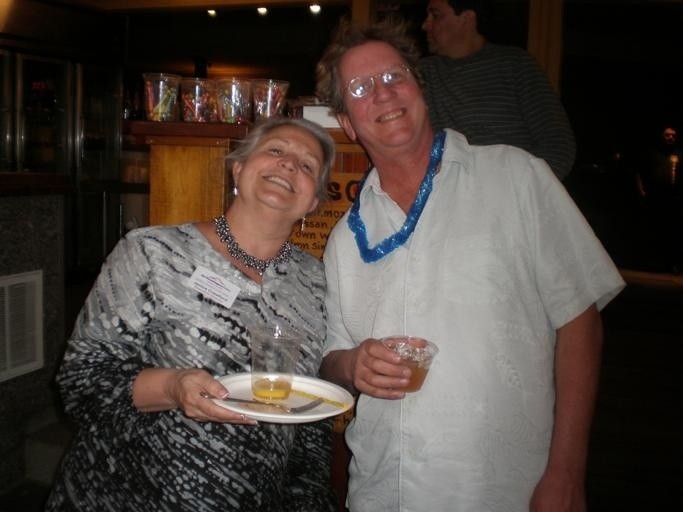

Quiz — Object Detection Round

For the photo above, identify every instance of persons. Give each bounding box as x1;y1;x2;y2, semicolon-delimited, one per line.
317;16;628;511
408;0;577;182
629;127;682;271
45;120;341;512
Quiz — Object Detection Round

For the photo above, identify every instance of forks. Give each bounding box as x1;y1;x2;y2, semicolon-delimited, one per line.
200;391;324;414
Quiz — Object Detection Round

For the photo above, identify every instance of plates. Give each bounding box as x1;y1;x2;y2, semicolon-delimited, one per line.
212;372;355;424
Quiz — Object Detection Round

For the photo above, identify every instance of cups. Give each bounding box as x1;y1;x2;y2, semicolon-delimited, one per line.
250;325;305;400
384;334;439;392
143;73;289;125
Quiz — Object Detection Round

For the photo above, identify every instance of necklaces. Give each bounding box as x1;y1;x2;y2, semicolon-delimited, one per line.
346;130;440;259
212;213;294;274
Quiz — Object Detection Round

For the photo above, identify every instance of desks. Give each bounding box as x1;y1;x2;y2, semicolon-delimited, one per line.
127;120;375;262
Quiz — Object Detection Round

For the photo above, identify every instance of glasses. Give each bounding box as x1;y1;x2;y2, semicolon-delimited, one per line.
343;62;413;99
